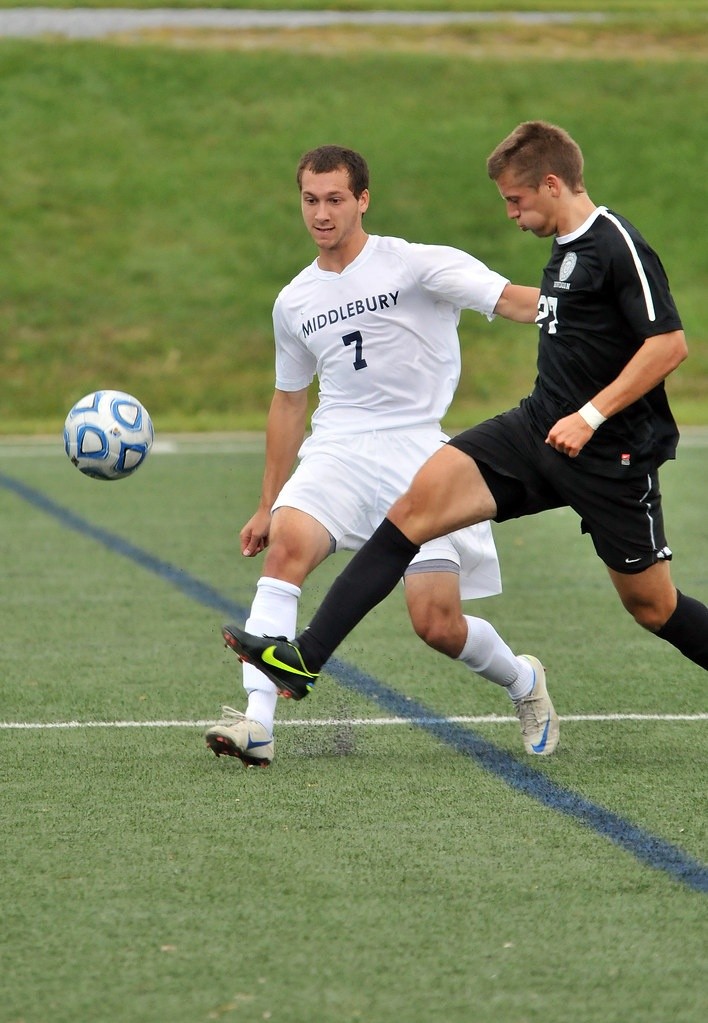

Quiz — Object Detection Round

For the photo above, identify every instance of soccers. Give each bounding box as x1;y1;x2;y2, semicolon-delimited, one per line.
63;389;154;481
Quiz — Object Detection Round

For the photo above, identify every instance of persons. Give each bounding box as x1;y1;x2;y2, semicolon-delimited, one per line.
204;141;561;769
222;119;708;704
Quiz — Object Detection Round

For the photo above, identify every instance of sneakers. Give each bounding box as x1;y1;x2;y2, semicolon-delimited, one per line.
207;719;276;766
220;623;317;699
507;653;557;759
